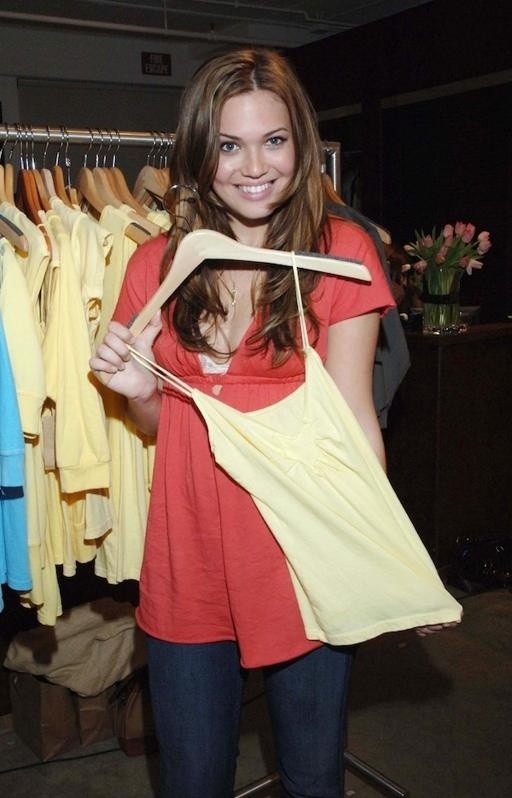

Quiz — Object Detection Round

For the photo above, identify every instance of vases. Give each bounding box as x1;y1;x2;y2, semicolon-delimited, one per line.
422;268;468;335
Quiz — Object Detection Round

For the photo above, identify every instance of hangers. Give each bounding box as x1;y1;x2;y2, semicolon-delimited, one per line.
99;183;373;386
0;122;170;266
321;140;392;245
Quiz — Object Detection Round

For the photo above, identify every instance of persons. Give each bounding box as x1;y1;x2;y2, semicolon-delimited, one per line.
86;42;398;796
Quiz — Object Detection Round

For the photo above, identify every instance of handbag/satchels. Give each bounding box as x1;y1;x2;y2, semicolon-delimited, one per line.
8;671;82;765
69;684;121;759
107;665;158;757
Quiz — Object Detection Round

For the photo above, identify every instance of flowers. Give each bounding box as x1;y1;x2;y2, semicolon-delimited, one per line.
402;222;492;335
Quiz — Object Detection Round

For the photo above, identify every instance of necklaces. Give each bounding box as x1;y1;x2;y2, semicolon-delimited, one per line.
225;263;258;307
